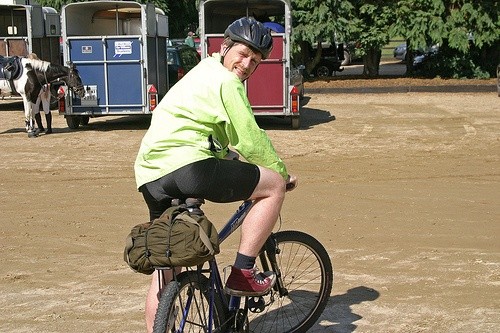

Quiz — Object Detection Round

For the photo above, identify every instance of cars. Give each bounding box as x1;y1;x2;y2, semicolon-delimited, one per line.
392;30;476;72
167;43;201;90
311;41;365;77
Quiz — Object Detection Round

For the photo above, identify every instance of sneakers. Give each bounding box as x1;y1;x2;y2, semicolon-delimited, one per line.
224;261;277;295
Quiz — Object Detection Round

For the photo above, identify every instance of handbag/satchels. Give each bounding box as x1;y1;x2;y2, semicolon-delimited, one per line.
124;205;220;275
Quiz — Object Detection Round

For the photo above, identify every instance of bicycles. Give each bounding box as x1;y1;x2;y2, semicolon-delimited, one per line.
153;158;334;333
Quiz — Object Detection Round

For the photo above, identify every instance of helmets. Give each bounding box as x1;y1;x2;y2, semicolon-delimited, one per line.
224;17;273;61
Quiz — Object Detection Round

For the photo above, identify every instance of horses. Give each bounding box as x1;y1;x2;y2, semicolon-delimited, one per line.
0;54;85;138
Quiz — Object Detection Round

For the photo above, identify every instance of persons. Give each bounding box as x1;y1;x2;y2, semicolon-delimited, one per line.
185;32;196;47
134;16;299;333
29;53;52;134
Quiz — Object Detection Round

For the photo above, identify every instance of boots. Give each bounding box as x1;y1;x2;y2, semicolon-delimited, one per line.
43;112;52;134
35;113;45;135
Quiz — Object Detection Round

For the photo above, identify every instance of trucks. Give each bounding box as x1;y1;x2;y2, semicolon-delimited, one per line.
60;0;169;132
198;0;306;130
0;0;62;101
